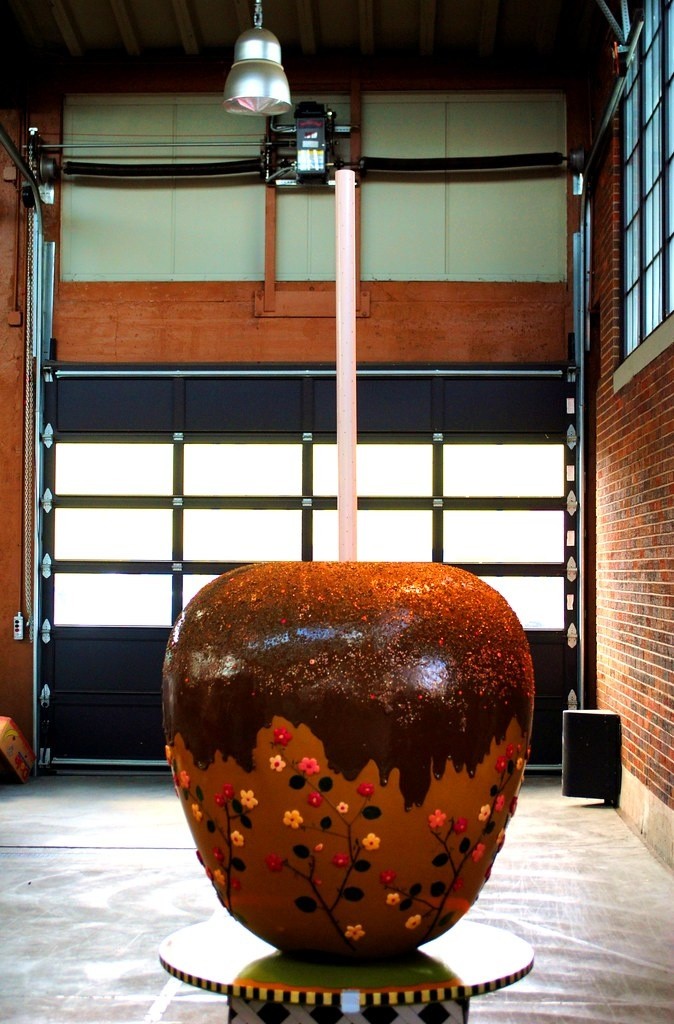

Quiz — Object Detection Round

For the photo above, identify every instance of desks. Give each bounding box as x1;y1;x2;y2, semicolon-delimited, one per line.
158;915;537;1024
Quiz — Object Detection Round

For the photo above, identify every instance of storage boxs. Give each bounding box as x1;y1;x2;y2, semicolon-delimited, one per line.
0;716;38;784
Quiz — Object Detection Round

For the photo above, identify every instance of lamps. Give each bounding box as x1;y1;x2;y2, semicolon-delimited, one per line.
222;0;293;115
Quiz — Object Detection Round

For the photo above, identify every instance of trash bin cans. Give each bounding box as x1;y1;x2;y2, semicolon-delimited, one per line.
560;708;622;797
